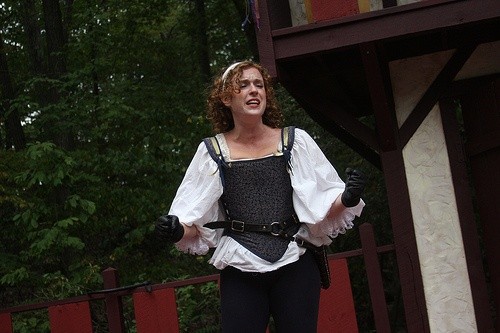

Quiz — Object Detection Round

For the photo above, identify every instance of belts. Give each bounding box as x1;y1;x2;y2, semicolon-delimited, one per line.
203;214;300;236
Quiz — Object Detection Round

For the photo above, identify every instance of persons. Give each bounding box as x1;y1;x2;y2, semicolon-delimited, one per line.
154;60;366;333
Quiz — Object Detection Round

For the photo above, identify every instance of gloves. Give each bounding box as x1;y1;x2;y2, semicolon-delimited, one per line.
158;215;185;244
341;168;365;208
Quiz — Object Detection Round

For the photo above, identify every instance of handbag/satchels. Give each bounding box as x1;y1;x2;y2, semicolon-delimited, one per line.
281;232;331;290
